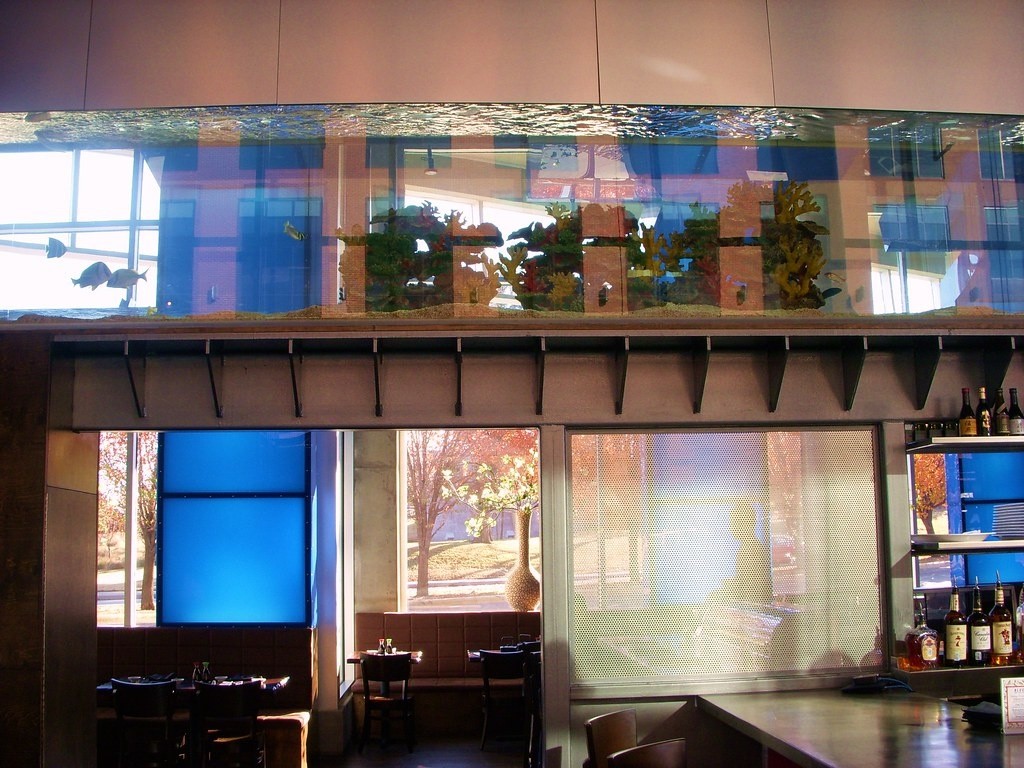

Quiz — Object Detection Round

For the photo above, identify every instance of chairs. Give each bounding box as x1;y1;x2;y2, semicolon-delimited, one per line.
545;709;685;768
357;652;418;754
111;677;264;768
479;649;531;752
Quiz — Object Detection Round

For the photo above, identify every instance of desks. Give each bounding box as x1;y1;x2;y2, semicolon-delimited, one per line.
696;686;1024;768
347;651;423;749
594;636;799;675
466;649;541;696
96;675;290;768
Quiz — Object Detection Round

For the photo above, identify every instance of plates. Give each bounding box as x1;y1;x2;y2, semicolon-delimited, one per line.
911;532;996;542
989;502;1024;540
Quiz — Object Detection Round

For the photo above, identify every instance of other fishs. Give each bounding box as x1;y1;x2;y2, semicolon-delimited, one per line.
45;237;69;258
34;130;68;153
283;221;308;241
72;262;148;291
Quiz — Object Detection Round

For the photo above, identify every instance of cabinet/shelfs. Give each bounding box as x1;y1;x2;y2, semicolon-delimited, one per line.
905;435;1024;619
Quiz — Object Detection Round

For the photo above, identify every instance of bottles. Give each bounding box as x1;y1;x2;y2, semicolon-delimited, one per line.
192;661;201;684
958;388;977;436
975;386;993;437
993;388;1011;436
1008;387;1024;436
968;575;992;667
943;574;968;669
904;591;940;672
378;639;385;654
989;569;1012;666
201;661;211;683
386;638;392;654
1017;581;1024;665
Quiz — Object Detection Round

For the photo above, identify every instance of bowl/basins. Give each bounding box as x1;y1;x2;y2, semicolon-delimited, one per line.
215;676;228;682
127;677;141;683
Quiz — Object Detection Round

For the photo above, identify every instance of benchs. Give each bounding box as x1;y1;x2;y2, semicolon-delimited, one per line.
97;627;311;768
351;601;782;738
